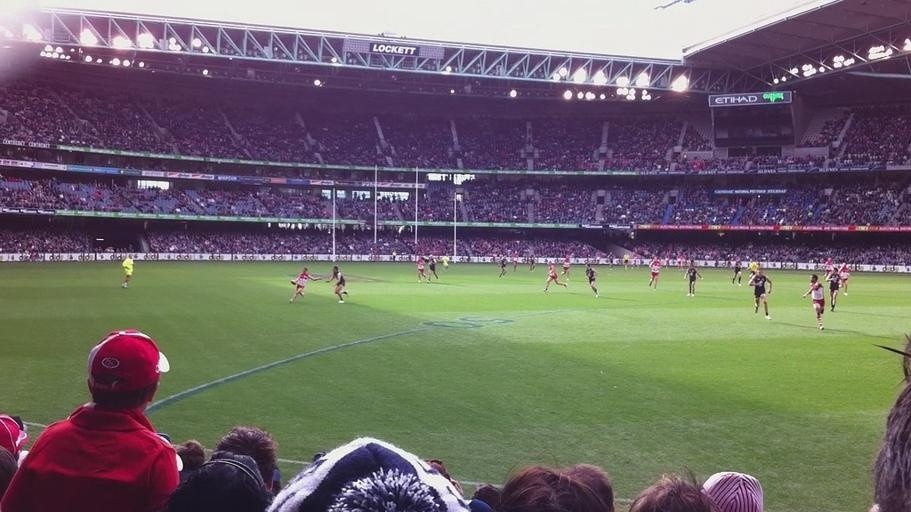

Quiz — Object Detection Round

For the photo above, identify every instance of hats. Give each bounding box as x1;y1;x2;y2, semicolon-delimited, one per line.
87;328;171;392
0;413;30;458
701;471;764;512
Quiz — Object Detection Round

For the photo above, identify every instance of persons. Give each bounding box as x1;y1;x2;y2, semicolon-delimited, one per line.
684;261;704;298
325;267;348;305
648;257;662;289
803;274;825;331
165;425;281;510
431;460;614;512
826;268;841;312
0;47;910;286
583;264;600;299
2;413;28;494
2;328;183;511
265;436;470;510
747;270;772;320
870;335;911;511
702;472;764;511
540;261;567;292
289;267;323;303
839;262;850;296
121;254;134;289
628;464;722;511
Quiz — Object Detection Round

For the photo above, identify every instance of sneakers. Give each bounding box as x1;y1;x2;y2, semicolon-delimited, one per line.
648;283;656;291
731;283;743;287
815;292;854;331
338;290;350;303
686;292;695;297
764;315;772;320
418;275;439;284
122;282;128;289
289;293;304;303
593;292;600;298
